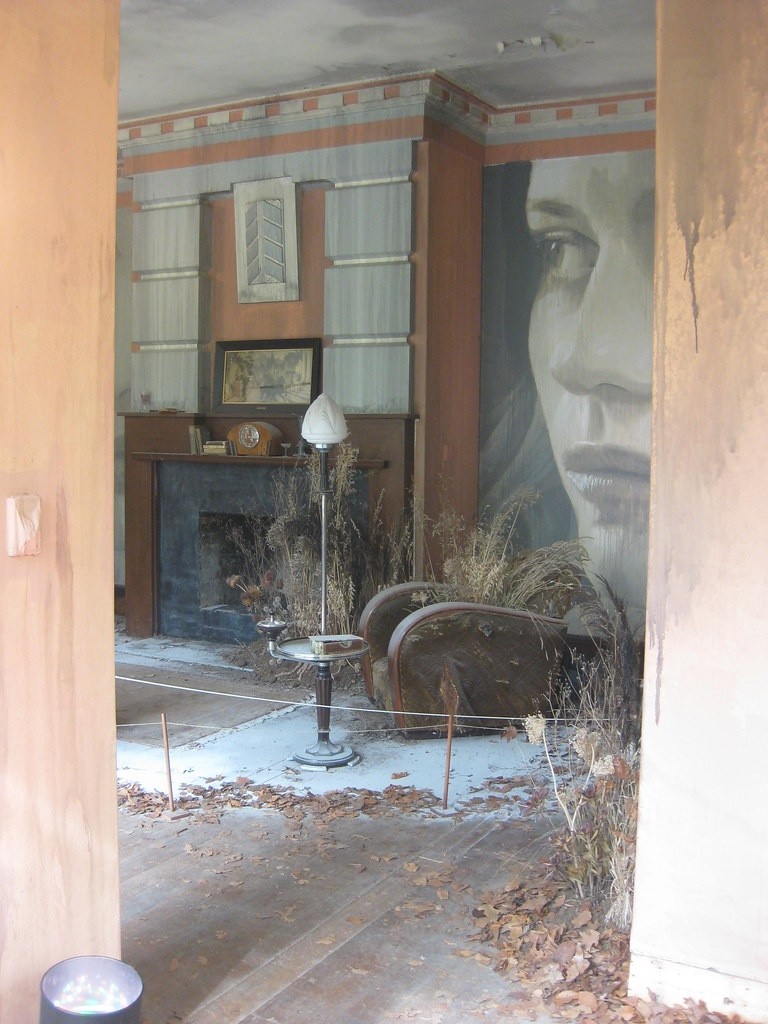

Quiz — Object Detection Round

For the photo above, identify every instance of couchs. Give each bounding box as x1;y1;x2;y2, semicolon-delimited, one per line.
358;581;569;740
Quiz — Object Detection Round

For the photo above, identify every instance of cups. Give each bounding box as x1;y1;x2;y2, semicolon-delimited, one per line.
40;956;143;1024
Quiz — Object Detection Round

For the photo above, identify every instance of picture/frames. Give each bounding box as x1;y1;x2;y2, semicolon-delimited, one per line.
214;337;321;414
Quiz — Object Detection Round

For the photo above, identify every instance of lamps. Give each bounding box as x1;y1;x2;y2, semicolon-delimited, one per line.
266;395;368;656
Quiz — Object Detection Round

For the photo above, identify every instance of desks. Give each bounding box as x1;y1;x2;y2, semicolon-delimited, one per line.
273;637;370;771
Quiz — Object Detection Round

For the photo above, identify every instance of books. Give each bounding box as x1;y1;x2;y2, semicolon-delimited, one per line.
188;425;230;455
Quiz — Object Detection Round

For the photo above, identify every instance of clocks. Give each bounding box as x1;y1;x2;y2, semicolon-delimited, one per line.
226;421;285;456
